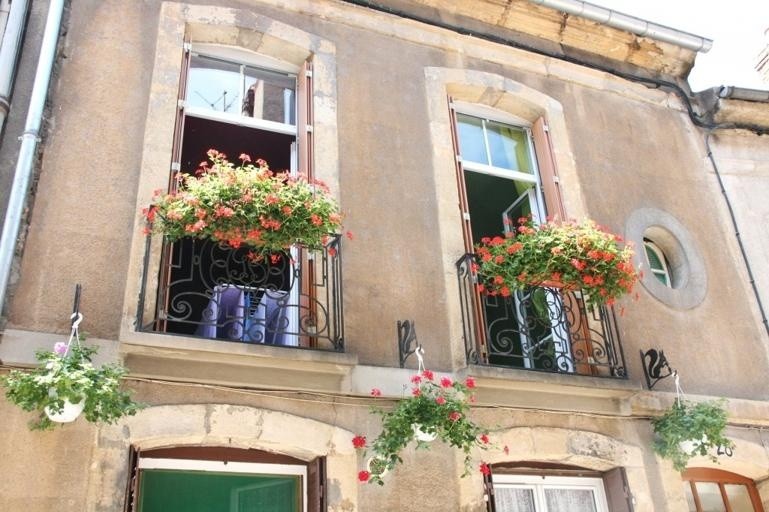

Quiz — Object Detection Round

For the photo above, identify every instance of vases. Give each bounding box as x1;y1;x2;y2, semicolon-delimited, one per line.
43;397;85;423
410;422;439;442
223;233;292;249
529;275;576;290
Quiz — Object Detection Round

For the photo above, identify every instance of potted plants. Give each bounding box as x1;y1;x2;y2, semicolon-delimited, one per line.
649;371;738;478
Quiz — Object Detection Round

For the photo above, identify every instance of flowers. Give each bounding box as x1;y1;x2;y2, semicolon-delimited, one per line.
471;215;645;318
135;150;354;267
351;368;511;487
1;332;151;433
